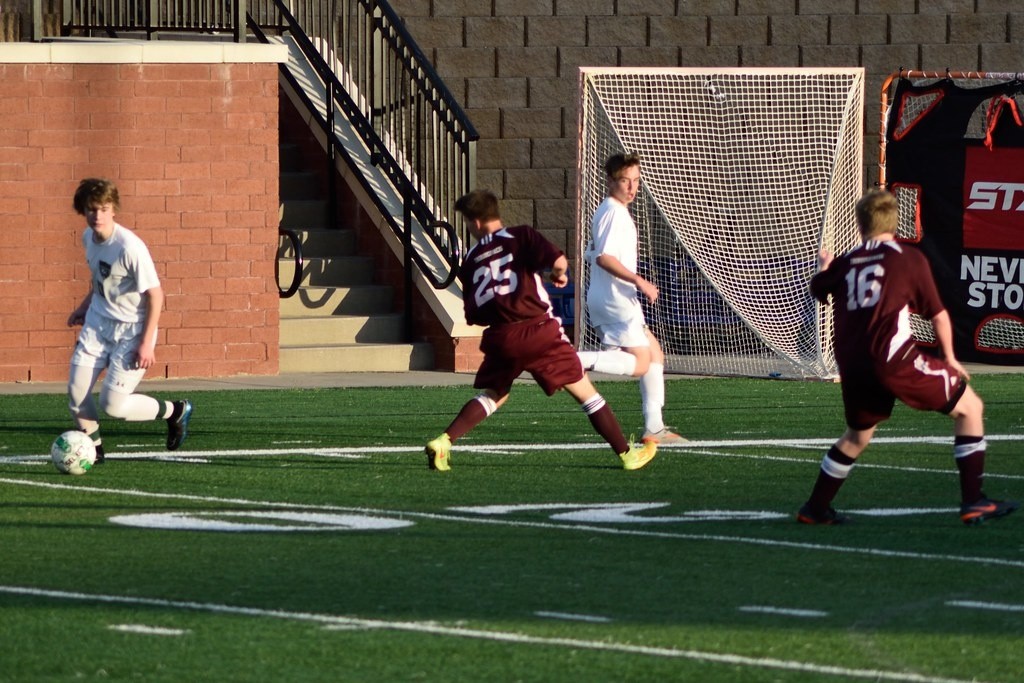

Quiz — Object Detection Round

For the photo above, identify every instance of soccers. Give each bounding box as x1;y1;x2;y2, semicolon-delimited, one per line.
50;429;97;476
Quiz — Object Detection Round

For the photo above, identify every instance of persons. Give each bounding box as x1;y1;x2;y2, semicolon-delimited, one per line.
66;178;193;465
576;151;689;444
427;190;657;471
797;187;1014;528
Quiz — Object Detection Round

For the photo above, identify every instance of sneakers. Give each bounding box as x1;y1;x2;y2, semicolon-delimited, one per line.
619;433;657;470
641;426;688;444
94;448;104;464
426;436;451;471
959;496;1020;525
166;398;193;450
796;503;851;524
